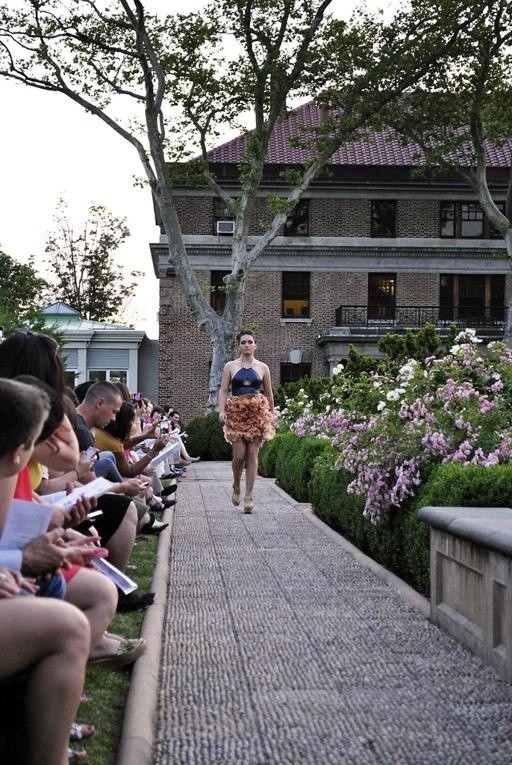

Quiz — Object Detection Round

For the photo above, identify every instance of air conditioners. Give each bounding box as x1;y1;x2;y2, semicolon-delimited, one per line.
215;219;237;235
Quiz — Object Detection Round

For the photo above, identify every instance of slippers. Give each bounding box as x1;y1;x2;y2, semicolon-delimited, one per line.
88;630;147;671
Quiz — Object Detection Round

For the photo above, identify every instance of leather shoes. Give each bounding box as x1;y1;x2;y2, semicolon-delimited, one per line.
148;497;178;512
144;522;169;535
116;587;158;613
155;484;177;496
138;513;155;534
159;455;201;480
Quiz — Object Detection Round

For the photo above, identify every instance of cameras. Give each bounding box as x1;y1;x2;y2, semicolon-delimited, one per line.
133;393;143;402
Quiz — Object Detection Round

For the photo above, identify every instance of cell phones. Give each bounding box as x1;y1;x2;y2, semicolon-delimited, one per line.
74;519;95;534
160;421;169;438
86;446;96;459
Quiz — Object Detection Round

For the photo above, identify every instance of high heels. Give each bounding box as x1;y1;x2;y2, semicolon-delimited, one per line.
231;482;241;506
243;495;255;514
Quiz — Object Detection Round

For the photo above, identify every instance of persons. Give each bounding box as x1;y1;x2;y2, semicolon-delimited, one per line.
218;328;277;516
1;328;200;765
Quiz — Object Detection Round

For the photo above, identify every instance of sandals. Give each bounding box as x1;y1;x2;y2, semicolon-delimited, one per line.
68;722;95;741
69;750;88;765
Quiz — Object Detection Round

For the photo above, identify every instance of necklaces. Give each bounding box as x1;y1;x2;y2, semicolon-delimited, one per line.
241;358;253;369
